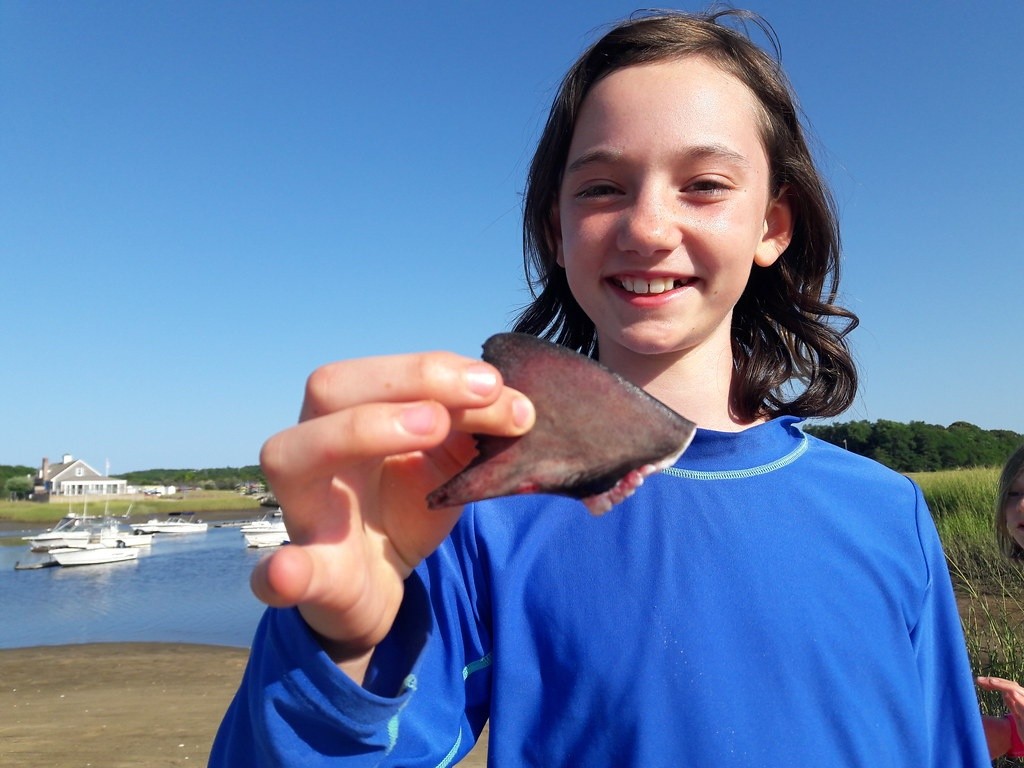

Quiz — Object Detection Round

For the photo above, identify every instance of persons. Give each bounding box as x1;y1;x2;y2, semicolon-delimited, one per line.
976;445;1024;761
207;4;994;768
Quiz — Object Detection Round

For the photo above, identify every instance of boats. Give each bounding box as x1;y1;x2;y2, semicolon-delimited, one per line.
240;512;291;547
48;521;139;568
273;507;282;517
215;519;252;528
130;516;208;533
22;493;156;552
